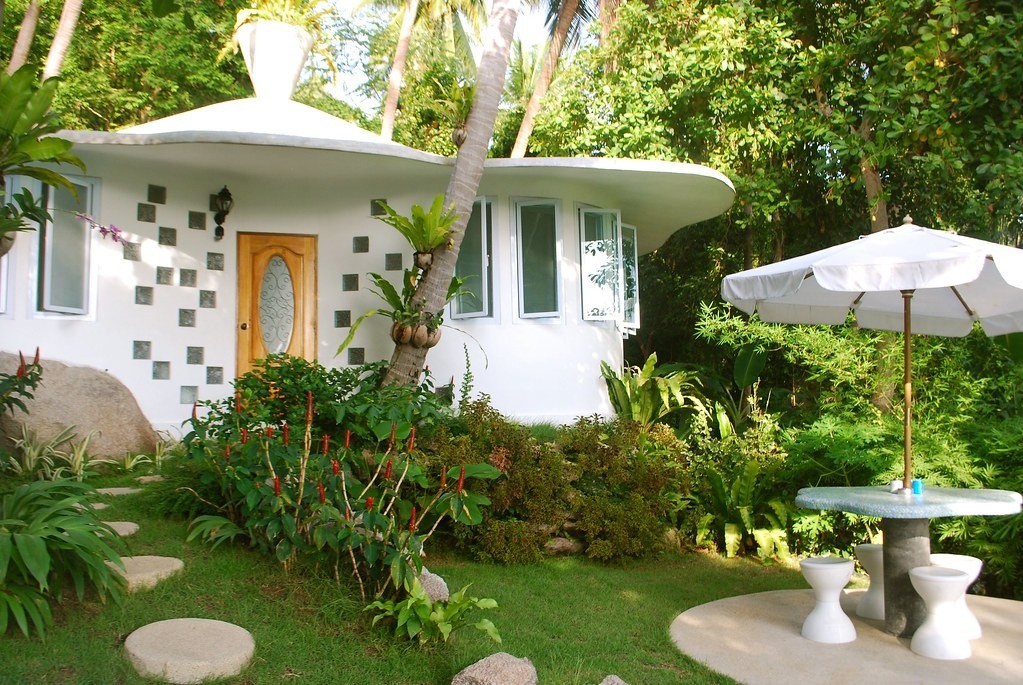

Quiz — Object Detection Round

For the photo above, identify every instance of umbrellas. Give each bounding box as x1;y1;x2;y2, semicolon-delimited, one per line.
722;214;1023;487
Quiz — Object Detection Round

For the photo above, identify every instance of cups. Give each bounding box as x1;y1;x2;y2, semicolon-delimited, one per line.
913;479;922;494
890;481;898;494
896;480;903;490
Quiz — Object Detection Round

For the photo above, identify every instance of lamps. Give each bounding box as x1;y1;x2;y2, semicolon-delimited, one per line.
212;185;234;239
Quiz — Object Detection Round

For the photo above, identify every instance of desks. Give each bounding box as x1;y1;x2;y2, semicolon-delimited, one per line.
795;485;1023;639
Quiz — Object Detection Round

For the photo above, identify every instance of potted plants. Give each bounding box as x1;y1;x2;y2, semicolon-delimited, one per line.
215;0;338;117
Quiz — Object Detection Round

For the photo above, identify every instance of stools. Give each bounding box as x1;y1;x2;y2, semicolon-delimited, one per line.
908;565;973;660
931;553;983;639
799;557;858;644
854;544;885;621
